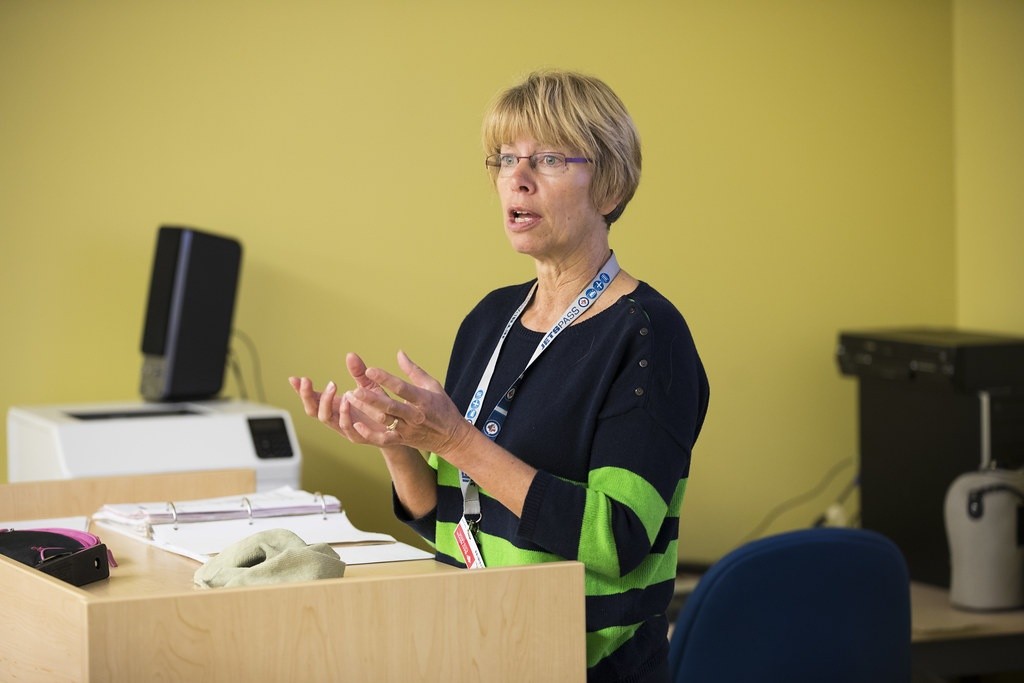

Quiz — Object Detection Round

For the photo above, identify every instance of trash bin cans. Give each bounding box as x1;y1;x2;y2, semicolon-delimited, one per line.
836;326;1023;588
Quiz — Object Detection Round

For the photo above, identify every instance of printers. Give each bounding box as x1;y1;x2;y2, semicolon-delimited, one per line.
5;405;303;497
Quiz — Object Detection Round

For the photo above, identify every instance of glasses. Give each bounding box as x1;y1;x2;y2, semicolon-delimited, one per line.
486;152;595;175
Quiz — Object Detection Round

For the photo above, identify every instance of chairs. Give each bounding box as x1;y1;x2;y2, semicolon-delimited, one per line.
654;525;914;683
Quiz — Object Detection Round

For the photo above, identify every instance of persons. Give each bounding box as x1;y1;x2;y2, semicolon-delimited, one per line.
293;75;711;682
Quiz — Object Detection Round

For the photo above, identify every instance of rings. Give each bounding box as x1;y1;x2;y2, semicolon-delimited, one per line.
386;417;399;431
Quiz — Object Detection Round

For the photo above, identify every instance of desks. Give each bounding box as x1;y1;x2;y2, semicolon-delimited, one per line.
0;467;587;683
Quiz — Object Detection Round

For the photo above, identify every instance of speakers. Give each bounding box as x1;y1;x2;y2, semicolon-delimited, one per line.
139;225;241;404
